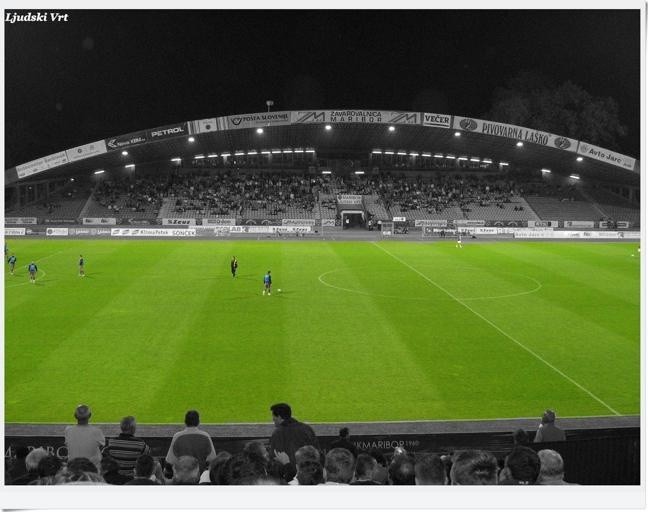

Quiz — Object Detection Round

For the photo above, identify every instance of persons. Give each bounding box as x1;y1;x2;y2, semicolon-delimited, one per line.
263;269;271;296
8;256;16;275
94;157;617;231
231;256;237;277
455;235;462;248
78;255;85;277
28;261;37;284
5;403;578;485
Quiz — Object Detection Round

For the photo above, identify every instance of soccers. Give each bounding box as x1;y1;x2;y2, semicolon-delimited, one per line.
276;288;282;295
